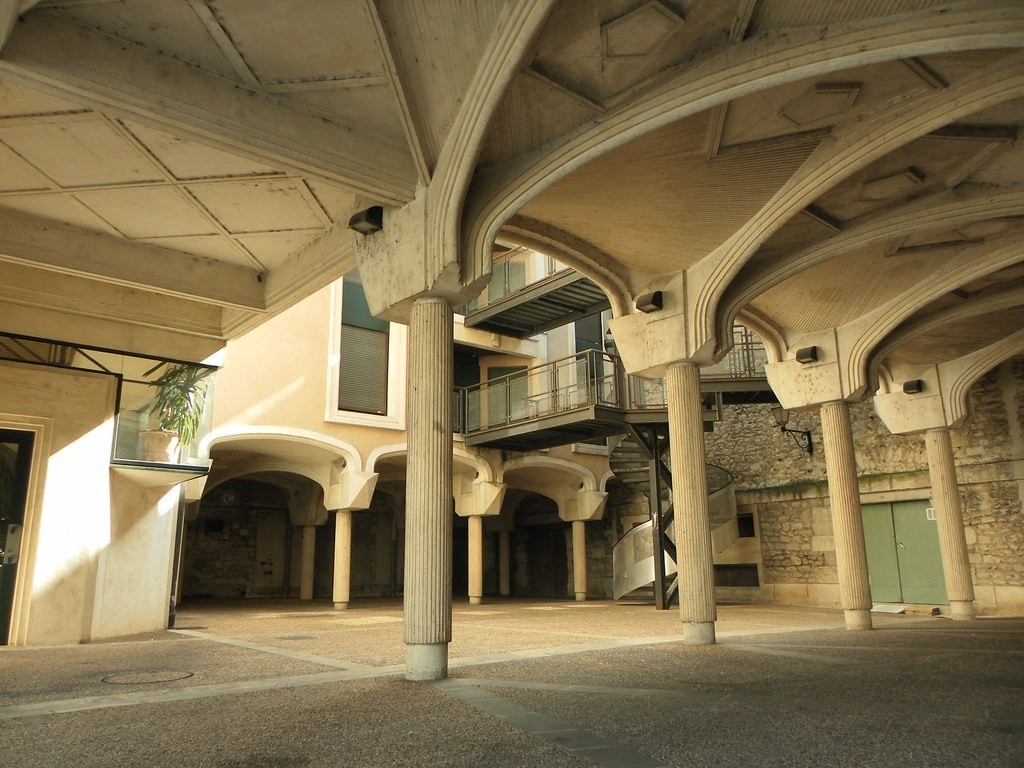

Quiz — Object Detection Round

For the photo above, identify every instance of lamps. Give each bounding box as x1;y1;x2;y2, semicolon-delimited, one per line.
770;407;813;455
796;346;818;364
903;380;922;394
635;291;663;314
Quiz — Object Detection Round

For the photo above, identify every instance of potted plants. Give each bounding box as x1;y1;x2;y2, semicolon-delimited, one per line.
138;360;210;462
